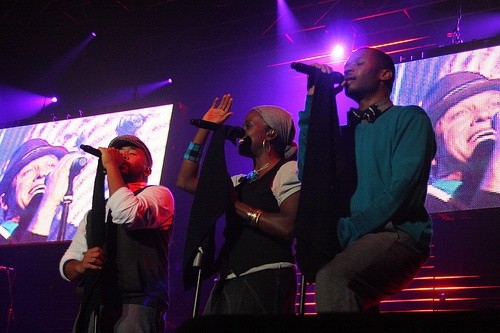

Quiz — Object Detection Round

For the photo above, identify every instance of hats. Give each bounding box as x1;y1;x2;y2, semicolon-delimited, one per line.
108;135;152;169
0;138;69;196
422;71;500;128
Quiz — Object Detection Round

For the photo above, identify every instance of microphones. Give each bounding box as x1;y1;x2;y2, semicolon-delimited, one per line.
190;118;245;138
81;144;123;170
69;157;87;178
290;62;345;84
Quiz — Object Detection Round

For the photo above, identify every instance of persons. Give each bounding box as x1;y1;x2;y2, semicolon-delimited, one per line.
421;70;500;213
0;137;79;245
297;46;437;315
58;135;176;333
176;94;302;316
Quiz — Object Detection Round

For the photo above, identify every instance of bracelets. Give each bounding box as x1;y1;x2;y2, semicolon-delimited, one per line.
247;207;263;231
184;142;205;162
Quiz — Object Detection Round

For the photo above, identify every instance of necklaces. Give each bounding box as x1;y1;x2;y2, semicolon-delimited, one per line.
246;157;278;181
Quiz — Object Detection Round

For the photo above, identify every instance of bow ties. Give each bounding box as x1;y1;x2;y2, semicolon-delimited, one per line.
347;104;381;125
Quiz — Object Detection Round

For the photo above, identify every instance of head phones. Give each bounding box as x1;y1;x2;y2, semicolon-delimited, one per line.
346;98;395;123
